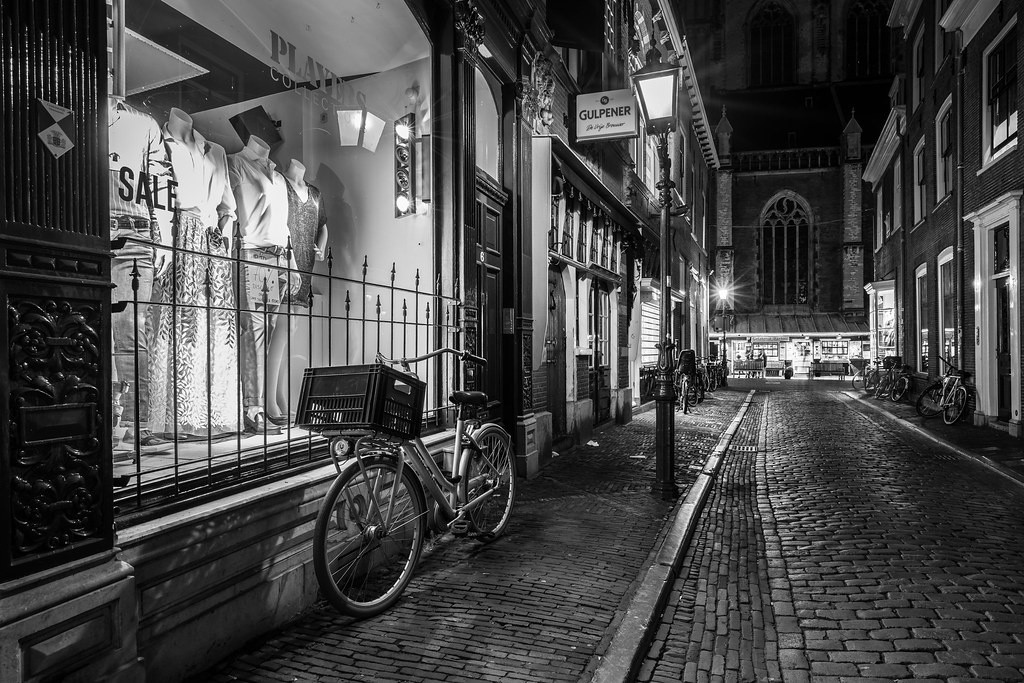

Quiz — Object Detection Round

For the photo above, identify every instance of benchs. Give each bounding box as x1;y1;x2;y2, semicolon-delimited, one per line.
733;360;764;378
766;361;784;377
809;362;846;381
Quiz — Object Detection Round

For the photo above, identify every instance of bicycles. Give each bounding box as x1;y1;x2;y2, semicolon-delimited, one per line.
851;357;913;403
916;355;972;425
642;354;732;414
293;346;519;620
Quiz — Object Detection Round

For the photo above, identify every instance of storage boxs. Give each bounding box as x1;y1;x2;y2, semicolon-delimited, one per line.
295;363;427;441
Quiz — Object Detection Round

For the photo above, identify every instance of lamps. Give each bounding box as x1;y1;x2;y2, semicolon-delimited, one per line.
652;207;689;218
394;113;432;219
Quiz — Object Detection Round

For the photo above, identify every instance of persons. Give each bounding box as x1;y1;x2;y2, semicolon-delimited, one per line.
145;107;244;434
108;96;174;454
266;158;327;427
225;136;302;435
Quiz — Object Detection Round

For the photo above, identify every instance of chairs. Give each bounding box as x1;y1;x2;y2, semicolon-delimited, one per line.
676;349;696;375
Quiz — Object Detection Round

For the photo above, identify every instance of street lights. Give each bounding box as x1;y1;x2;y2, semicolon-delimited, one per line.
628;19;688;499
718;289;729;364
